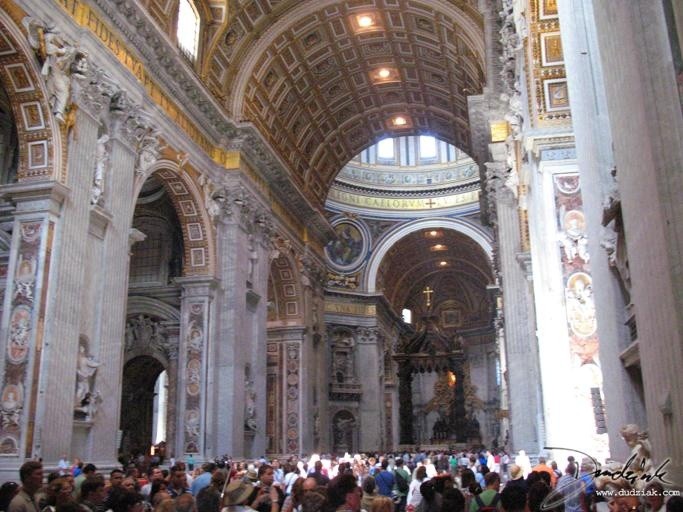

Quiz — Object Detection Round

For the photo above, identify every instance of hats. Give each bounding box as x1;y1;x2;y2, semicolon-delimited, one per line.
213;470;259;506
511;465;524;481
48;470;70;483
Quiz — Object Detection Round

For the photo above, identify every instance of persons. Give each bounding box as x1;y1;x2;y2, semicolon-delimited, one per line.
0;423;683;512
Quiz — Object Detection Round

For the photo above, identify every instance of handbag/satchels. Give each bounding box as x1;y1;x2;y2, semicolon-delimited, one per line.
395;470;408;492
